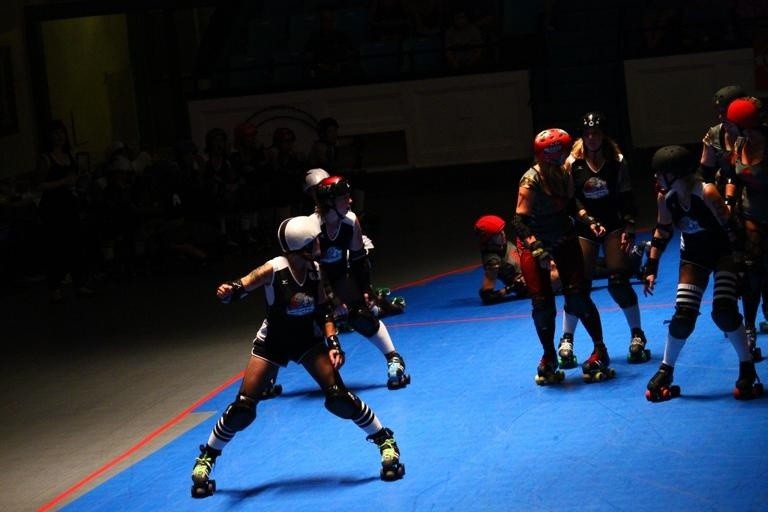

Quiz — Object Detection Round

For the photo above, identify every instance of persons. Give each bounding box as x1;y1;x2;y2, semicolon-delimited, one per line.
190;216;399;482
557;112;649;359
720;94;768;349
259;173;405;391
698;85;748;209
512;129;610;374
444;10;484;77
642;145;757;391
471;214;657;306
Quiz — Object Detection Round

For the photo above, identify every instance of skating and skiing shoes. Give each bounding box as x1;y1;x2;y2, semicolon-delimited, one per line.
533;352;566;386
624;328;652;364
730;359;764;399
383;351;412;390
643;362;682;401
366;425;407;480
187;442;223;499
556;332;580;368
581;344;617;385
742;327;764;363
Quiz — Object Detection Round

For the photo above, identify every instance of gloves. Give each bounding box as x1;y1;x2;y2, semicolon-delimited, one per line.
639;257;660;285
360;284;378;303
503;275;524;295
527;240;556;265
732;260;752;295
621;220;637;244
580;210;605;230
217;283;235;305
327;333;346;368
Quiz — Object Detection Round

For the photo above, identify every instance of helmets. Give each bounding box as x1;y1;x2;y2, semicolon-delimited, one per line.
314;175;354;201
473;213;507;245
713;84;748;108
578;109;610;134
726;95;767;130
300;165;331;194
531;128;574;163
276;216;324;253
648;143;697;176
